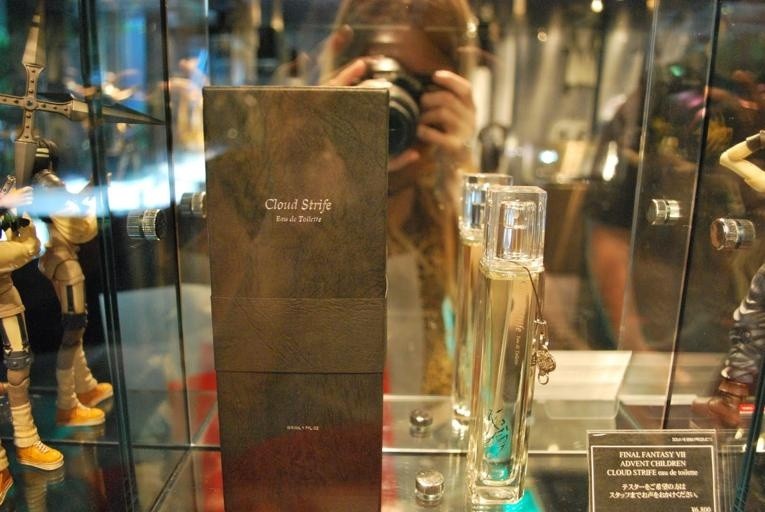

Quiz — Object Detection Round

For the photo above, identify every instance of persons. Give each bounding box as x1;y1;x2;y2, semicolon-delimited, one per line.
318;0;479;401
691;125;765;429
580;0;765;351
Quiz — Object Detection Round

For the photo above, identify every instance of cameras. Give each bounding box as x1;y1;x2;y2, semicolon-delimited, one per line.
350;57;447;160
